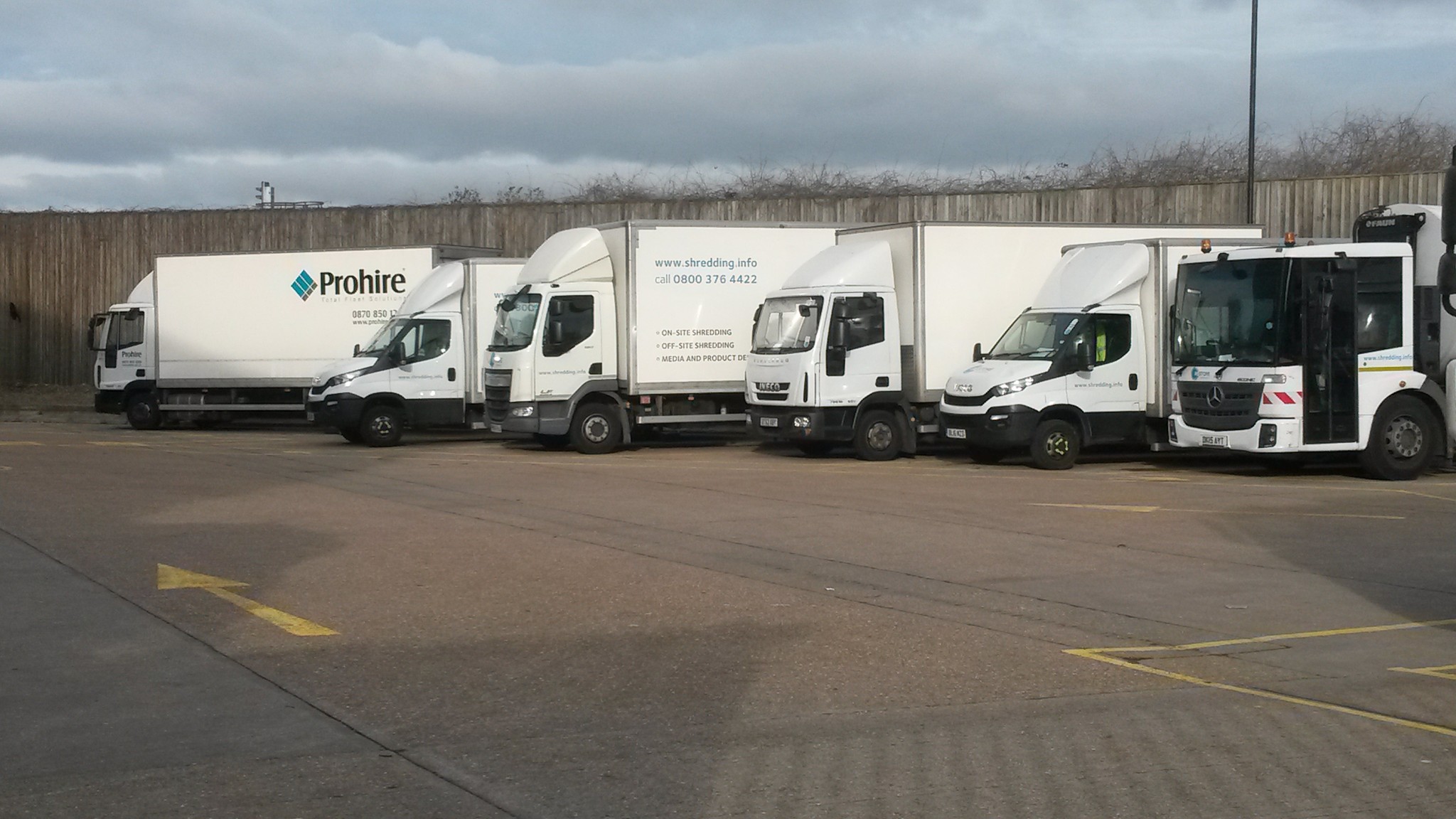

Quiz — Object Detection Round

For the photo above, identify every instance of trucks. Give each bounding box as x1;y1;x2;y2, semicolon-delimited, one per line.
745;219;1267;463
1167;203;1456;482
939;238;1354;470
480;220;895;454
85;243;502;430
304;257;529;447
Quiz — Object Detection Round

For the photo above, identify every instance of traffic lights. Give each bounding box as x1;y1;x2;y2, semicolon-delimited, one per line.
255;181;271;209
